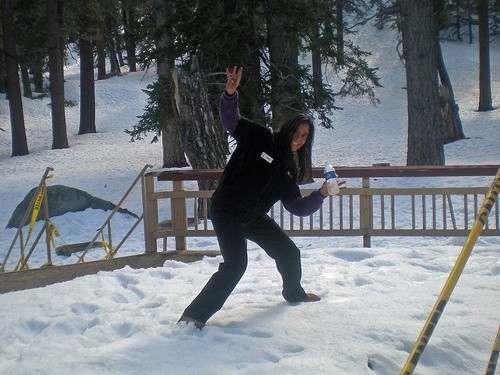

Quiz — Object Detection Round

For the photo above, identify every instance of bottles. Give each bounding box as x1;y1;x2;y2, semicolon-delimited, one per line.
324;161;339;195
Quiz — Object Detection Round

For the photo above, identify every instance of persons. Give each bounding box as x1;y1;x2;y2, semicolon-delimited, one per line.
176;66;347;325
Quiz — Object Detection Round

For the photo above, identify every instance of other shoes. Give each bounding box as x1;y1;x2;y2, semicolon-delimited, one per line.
306;293;321;301
176;315;206;330
